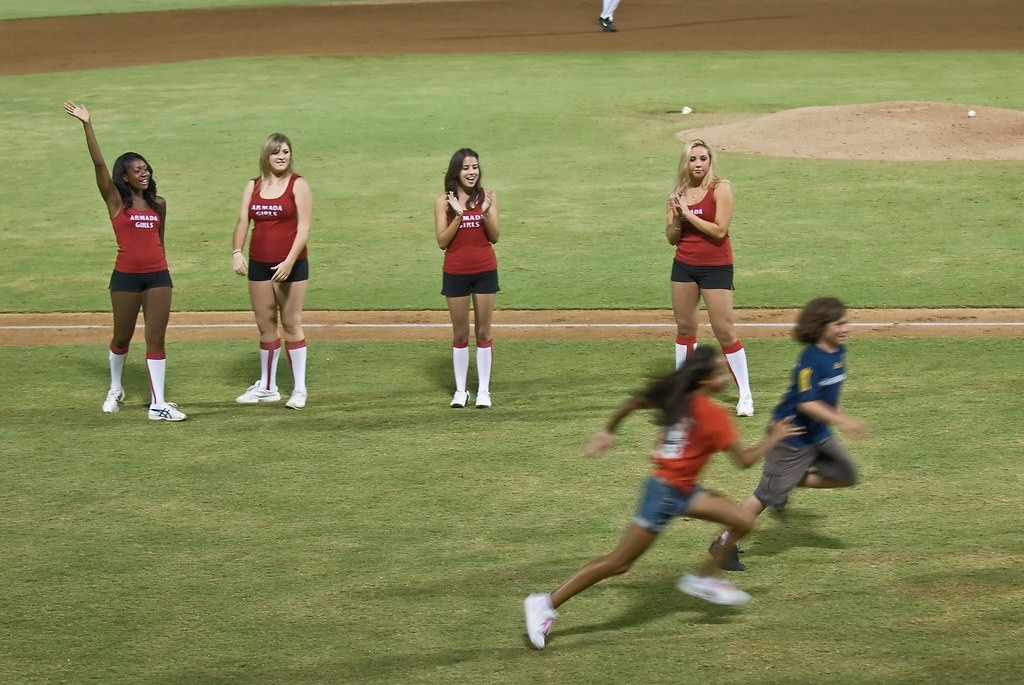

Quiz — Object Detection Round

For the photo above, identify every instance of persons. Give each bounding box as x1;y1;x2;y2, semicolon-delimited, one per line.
709;297;867;571
667;139;754;417
523;346;806;651
232;134;312;411
434;148;500;406
597;0;621;31
63;100;188;421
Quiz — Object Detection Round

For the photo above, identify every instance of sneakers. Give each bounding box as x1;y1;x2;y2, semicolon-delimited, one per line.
235;380;281;403
149;402;187;421
708;540;747;570
682;574;744;605
524;595;556;649
475;392;492;407
285;391;307;408
736;396;754;416
102;387;126;413
598;17;616;31
451;390;470;408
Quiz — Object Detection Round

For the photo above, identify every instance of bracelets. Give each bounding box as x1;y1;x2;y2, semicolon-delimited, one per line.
232;251;241;256
